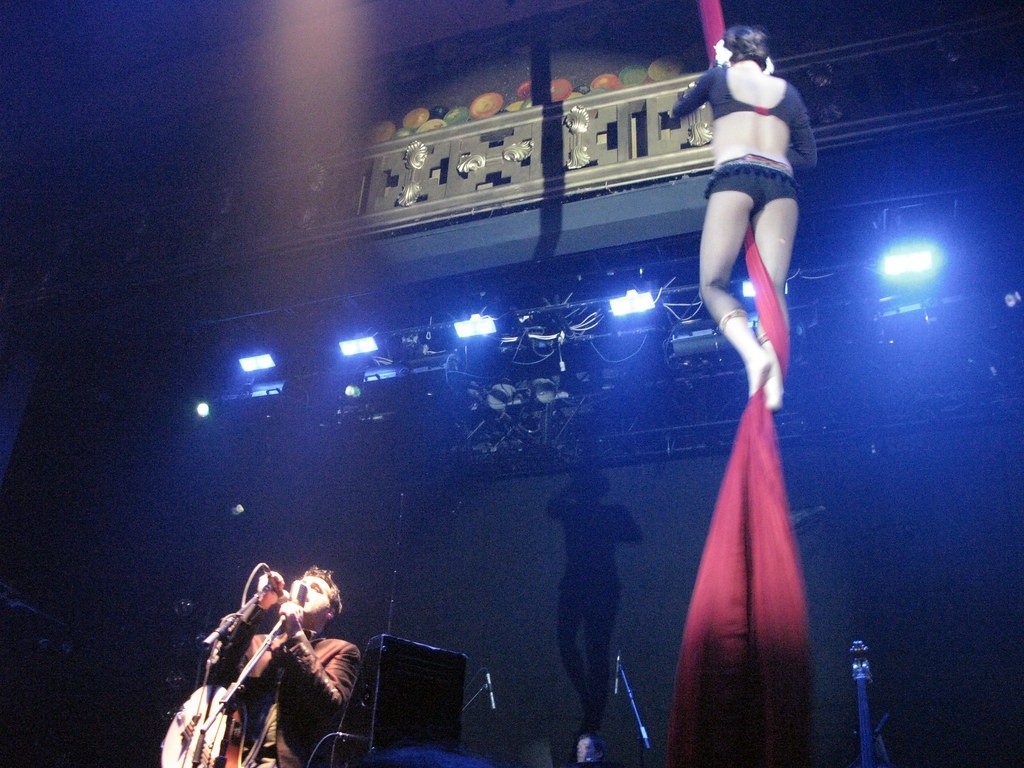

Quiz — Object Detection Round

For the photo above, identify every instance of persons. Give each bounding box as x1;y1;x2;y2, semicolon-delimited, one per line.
211;564;362;768
664;26;814;407
576;733;605;762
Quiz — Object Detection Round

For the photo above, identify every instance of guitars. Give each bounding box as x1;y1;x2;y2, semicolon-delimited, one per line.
159;684;244;768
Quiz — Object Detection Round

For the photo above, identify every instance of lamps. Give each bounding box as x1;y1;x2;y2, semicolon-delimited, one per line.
453;313;497;338
609;287;655;318
338;331;381;357
238;351;276;373
740;277;789;298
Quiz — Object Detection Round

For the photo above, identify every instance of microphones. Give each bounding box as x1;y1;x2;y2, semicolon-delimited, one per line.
486;670;496;710
292;582;308;607
263;565;290;605
615;651;621;694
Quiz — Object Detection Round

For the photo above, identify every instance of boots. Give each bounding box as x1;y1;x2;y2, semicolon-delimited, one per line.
719;309;771;397
757;332;785;412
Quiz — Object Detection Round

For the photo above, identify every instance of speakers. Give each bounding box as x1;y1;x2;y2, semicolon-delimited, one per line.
355;633;467;754
